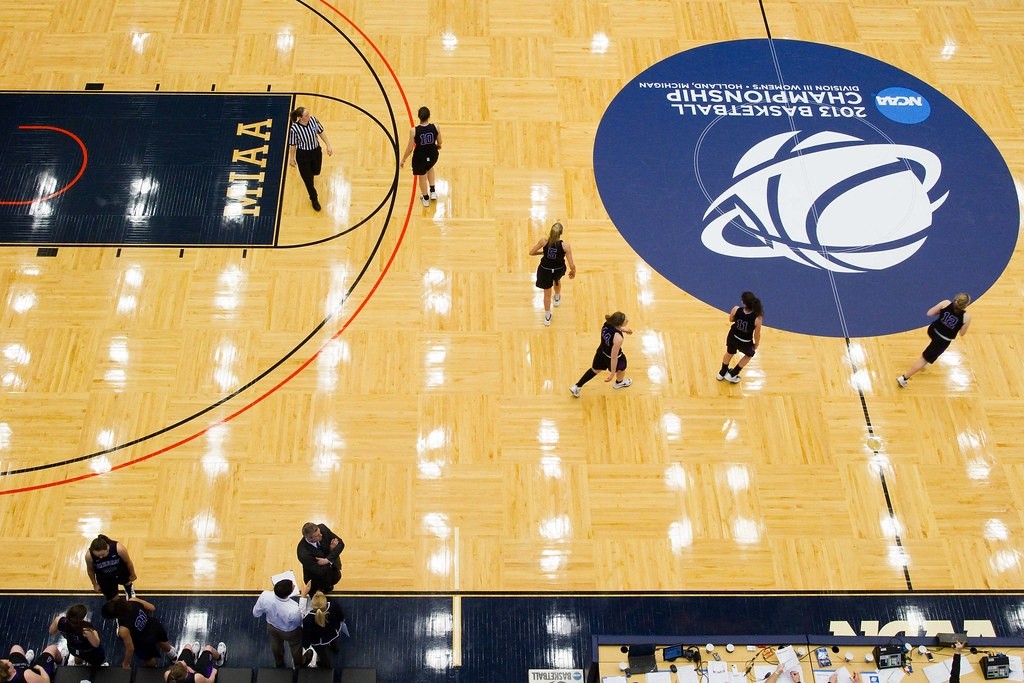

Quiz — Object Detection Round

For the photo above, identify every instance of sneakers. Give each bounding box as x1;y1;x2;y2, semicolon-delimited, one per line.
716;367;741;383
25;649;35;664
569;383;582;398
613;378;632;389
167;645;177;661
57;646;69;666
123;584;136;601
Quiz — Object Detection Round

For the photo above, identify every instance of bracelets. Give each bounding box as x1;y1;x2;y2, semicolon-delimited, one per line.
774;672;780;677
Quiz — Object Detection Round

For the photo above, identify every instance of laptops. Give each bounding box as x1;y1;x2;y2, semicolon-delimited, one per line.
628;644;658;674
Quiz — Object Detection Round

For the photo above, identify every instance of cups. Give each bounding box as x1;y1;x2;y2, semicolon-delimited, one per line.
726;643;735;653
618;662;627;672
918;646;927;655
705;643;715;653
845;653;853;662
865;654;874;663
903;643;913;653
798;647;806;657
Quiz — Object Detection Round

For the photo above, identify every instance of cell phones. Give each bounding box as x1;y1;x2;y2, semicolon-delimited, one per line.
926;651;934;661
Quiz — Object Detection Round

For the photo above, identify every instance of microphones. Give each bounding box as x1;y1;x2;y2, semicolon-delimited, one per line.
687;645;700;662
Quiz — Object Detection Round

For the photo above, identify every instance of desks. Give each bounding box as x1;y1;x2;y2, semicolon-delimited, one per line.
590;635;1024;681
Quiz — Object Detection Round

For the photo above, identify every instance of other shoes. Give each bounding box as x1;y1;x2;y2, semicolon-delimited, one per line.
101;662;109;666
192;642;200;664
311;199;321;211
896;375;907;388
216;642;226;666
304;649;313;667
420;194;429;207
544;313;552;326
553;292;561;307
430;191;438;199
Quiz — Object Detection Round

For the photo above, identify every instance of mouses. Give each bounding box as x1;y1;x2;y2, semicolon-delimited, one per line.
670;664;677;673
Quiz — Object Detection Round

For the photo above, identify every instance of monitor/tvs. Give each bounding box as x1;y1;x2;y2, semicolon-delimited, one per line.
663;643;683;662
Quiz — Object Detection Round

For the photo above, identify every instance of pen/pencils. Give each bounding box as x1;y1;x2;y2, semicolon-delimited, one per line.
790;671;799;678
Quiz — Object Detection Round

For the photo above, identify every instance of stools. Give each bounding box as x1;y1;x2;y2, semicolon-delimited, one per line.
56;666;377;683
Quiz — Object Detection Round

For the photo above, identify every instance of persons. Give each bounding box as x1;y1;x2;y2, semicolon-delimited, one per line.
400;106;442;207
301;590;346;664
828;669;864;683
48;604;110;667
528;222;576;327
163;642;227;683
288;107;332;212
948;639;966;683
764;662;802;683
297;522;345;599
253;580;303;672
895;293;972;388
716;291;763;383
568;311;633;398
86;534;137;602
101;597;175;671
0;644;70;683
